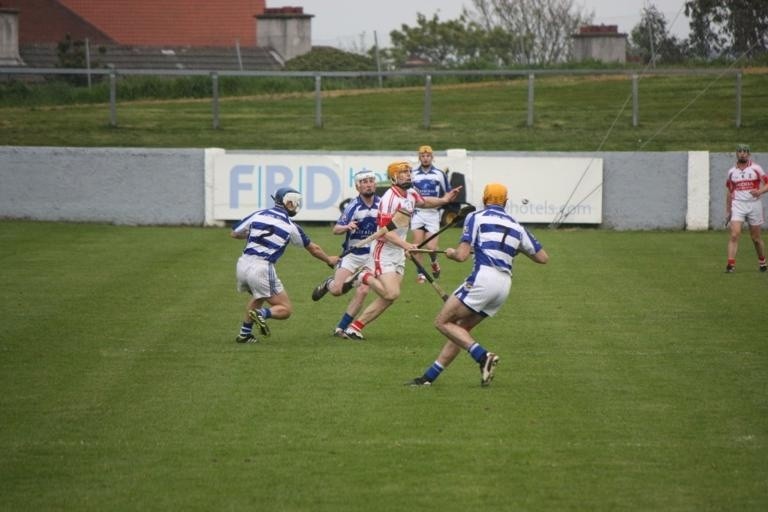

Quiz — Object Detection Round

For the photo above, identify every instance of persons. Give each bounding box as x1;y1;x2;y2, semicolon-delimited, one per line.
408;146;452;285
724;143;768;274
312;169;397;336
343;162;462;341
405;183;548;386
231;188;341;344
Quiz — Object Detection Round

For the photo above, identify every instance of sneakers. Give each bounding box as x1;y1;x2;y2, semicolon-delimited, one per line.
480;351;500;387
760;265;768;272
313;264;371;340
727;264;736;273
236;308;270;343
417;260;440;283
405;377;432;384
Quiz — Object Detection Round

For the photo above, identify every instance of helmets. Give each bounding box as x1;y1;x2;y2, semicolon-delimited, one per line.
736;143;750;151
271;186;303;217
483;183;507;205
419;145;433;160
353;161;412;189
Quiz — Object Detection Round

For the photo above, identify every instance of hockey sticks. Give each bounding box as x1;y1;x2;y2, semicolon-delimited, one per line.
408;249;475;255
411;255;449;302
338;207;411;258
416;206;476;248
